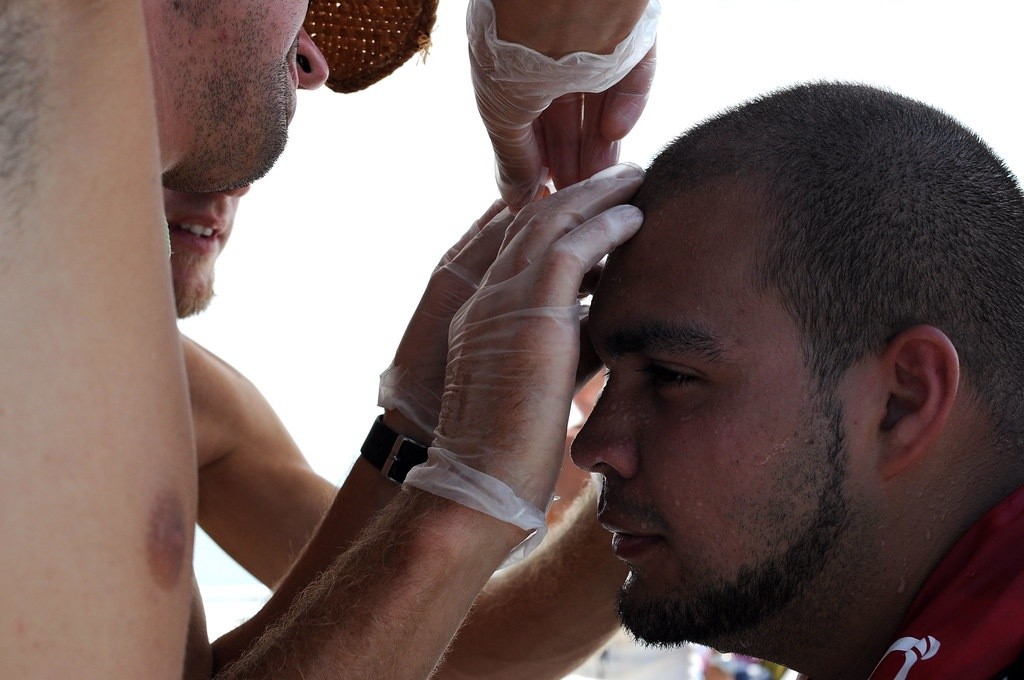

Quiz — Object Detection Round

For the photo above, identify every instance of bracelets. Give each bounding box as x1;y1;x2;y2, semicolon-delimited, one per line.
360;417;429;489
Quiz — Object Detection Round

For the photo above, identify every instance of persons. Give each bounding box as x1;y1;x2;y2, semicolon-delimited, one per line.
0;0;657;680
570;77;1023;679
